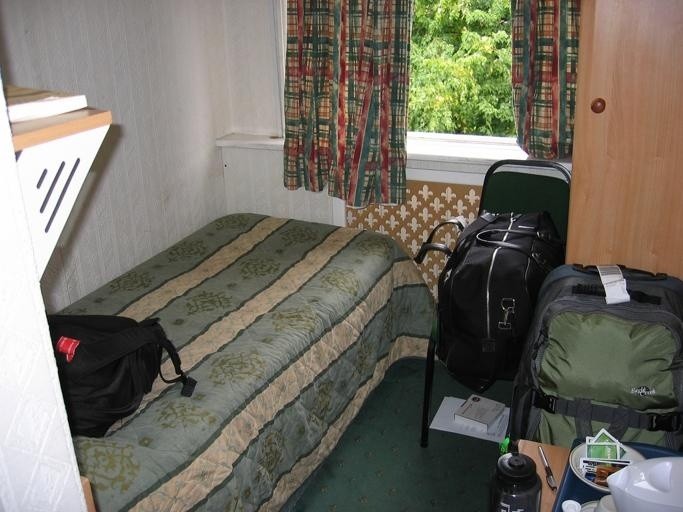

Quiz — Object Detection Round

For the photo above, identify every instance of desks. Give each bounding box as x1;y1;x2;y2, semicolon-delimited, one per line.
516;438;571;512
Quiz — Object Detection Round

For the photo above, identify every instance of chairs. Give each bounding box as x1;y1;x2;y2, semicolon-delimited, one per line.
419;160;570;449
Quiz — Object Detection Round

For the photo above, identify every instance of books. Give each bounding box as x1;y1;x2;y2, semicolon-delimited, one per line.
452;393;506;433
2;76;89;124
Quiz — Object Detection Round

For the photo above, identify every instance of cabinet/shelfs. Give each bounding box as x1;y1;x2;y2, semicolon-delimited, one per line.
566;0;683;282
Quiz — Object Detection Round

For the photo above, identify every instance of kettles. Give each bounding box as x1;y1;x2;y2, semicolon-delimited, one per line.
605;455;683;512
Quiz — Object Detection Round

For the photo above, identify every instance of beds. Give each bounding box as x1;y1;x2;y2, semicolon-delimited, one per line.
52;214;396;512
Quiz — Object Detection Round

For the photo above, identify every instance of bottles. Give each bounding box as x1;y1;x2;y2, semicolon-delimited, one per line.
490;452;542;512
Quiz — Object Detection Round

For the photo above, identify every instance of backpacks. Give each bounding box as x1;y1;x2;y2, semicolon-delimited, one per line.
45;312;202;443
505;253;681;451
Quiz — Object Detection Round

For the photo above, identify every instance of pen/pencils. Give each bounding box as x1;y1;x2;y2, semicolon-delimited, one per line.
538;445;558;491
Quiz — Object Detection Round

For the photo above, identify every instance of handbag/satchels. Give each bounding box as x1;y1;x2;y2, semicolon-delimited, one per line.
433;204;567;395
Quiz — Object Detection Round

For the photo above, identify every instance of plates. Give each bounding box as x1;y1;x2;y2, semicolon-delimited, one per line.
568;438;647;493
580;500;600;512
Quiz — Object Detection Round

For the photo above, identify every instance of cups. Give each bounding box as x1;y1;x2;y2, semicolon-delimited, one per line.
594;493;617;512
